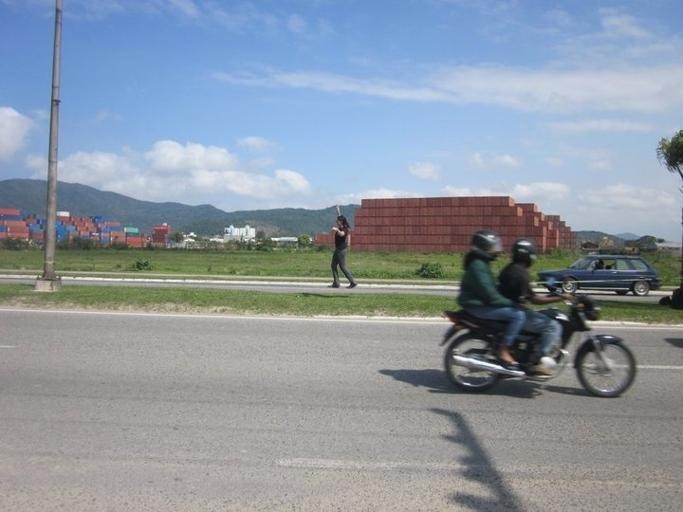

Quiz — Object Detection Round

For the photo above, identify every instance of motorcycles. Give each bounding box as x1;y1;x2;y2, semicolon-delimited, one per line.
438;283;635;397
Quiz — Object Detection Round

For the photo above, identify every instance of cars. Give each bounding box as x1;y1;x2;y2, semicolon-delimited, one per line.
536;254;662;297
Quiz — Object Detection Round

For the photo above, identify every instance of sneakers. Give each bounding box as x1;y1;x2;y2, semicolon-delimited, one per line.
528;365;552;375
329;285;339;288
497;349;518;365
347;283;355;288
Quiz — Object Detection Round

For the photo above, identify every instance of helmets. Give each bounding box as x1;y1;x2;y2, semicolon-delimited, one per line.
469;230;503;257
512;239;537;266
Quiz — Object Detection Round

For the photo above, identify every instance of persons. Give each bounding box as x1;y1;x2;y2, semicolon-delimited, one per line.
459;229;525;369
499;240;572;377
329;204;358;289
